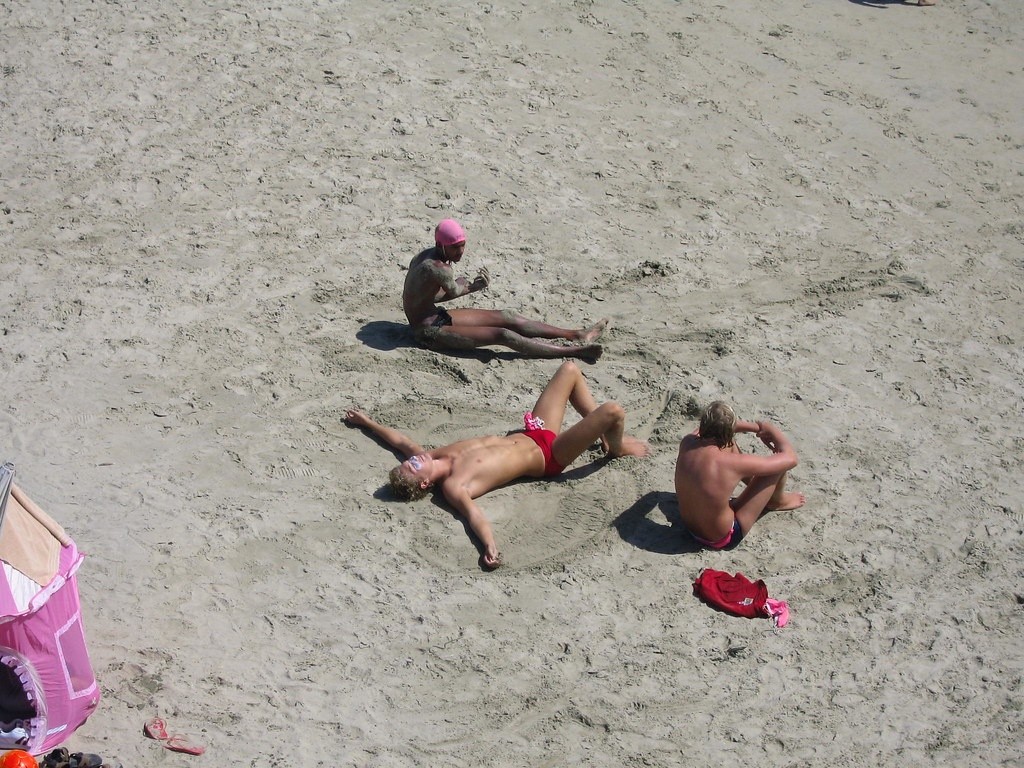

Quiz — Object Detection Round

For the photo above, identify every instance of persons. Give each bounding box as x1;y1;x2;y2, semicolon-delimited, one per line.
340;359;650;568
403;217;609;362
674;400;806;551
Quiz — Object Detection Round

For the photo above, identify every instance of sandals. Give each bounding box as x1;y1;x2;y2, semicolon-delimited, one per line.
40;747;123;768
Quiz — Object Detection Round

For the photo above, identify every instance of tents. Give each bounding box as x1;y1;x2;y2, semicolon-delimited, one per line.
0;464;99;760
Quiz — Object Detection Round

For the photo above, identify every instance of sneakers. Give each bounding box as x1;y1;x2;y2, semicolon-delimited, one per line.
0;719;33;751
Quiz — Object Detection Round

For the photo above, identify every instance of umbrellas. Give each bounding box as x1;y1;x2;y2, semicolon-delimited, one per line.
0;460;15;533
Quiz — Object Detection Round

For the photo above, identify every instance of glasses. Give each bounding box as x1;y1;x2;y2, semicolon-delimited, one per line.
725;404;736;429
410;456;421;470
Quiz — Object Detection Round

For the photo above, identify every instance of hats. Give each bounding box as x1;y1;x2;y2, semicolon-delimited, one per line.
435;219;465;245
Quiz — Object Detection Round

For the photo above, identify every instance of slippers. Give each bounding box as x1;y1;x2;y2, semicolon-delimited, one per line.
164;734;205;755
144;716;168;740
